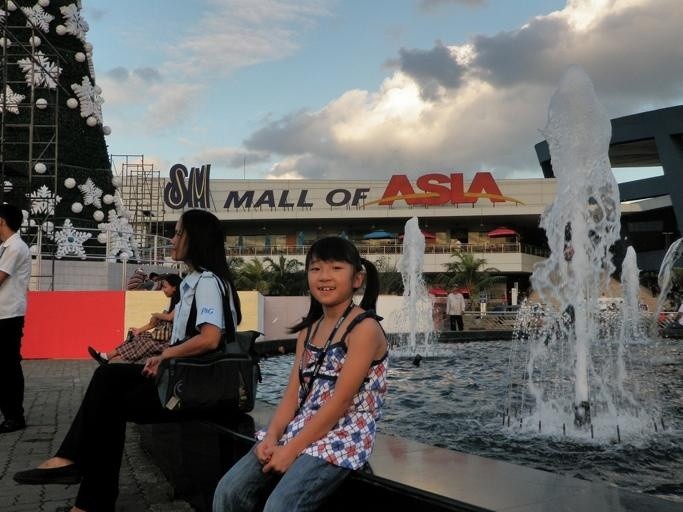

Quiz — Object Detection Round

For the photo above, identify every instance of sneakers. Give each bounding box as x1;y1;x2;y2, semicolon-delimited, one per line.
0;416;25;434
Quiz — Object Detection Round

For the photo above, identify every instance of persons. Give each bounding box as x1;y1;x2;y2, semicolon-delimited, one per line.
149;272;175;290
607;303;619;312
672;292;683;325
12;209;242;512
212;236;389;512
88;274;183;365
0;205;32;435
446;283;466;331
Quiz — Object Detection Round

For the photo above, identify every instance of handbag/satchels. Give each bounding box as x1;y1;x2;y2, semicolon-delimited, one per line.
157;331;262;413
150;321;173;344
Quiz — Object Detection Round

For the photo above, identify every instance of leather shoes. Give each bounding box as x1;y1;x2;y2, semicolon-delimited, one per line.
13;464;80;484
56;506;73;512
88;347;109;366
